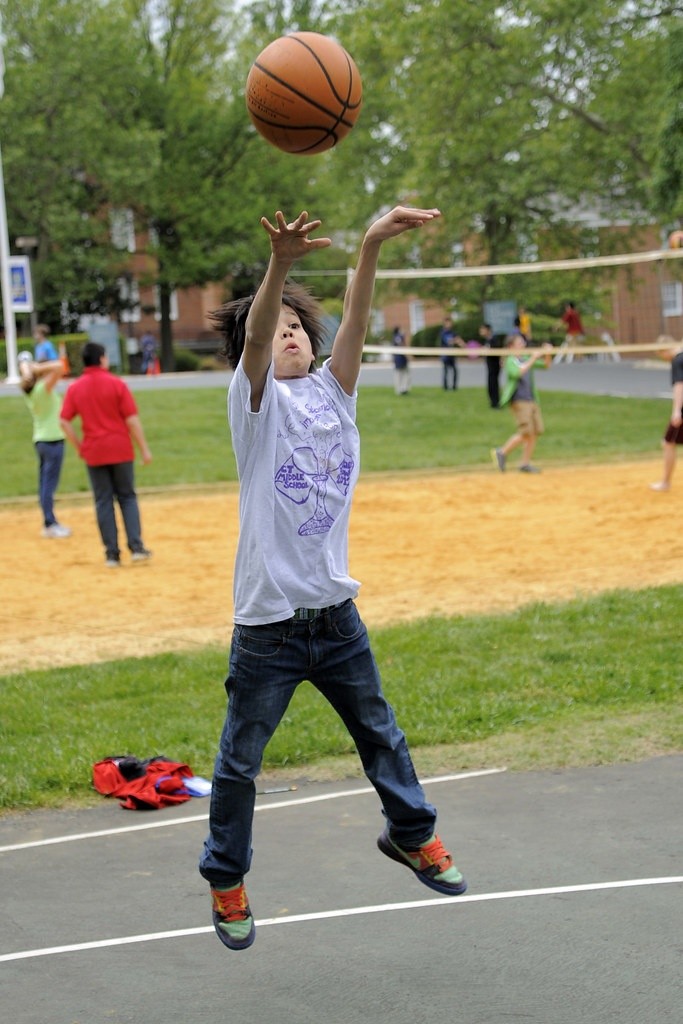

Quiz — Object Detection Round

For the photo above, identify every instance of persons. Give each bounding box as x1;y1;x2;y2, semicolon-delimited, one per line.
490;334;554;473
392;301;583;408
59;344;156;566
16;327;71;535
651;334;683;492
200;206;468;949
126;329;157;372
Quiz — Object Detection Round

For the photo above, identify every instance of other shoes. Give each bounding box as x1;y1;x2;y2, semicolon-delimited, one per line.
519;464;541;473
131;549;152;560
105;553;120;568
650;482;670;490
491;448;507;474
44;523;71;538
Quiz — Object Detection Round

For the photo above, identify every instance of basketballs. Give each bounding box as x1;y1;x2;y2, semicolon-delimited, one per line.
248;30;362;155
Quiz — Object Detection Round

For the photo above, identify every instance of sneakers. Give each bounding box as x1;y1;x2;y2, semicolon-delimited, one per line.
376;828;467;896
209;883;255;951
